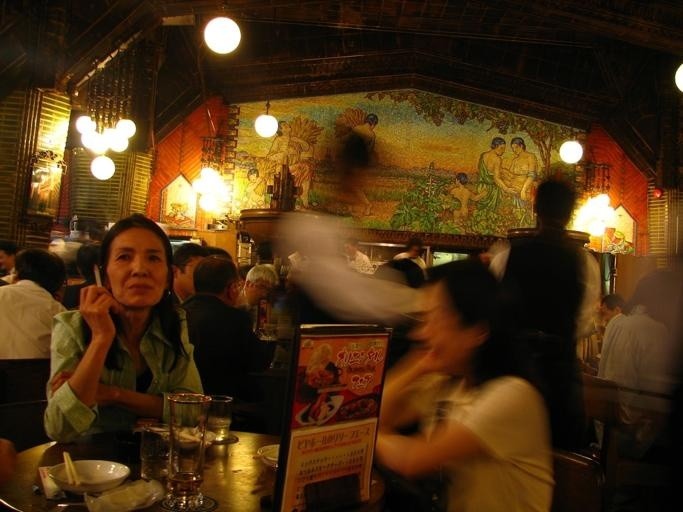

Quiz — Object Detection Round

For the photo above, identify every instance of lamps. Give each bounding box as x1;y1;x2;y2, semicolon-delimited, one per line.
75;51;136;154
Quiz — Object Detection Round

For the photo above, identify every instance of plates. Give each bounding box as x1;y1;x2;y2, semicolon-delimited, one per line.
47;460;131;492
296;395;344;425
257;445;280;468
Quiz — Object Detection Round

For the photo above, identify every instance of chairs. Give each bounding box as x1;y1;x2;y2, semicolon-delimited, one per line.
2;359;50;447
544;434;609;512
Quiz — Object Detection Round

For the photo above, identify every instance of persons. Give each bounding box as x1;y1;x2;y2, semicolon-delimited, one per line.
1;113;682;510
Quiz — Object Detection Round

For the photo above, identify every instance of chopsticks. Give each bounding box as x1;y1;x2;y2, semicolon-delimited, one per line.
62;451;81;486
252;449;277;459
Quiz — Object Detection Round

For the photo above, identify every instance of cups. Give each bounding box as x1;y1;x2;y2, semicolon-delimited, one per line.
140;424;179;479
205;395;234;441
166;393;212;508
